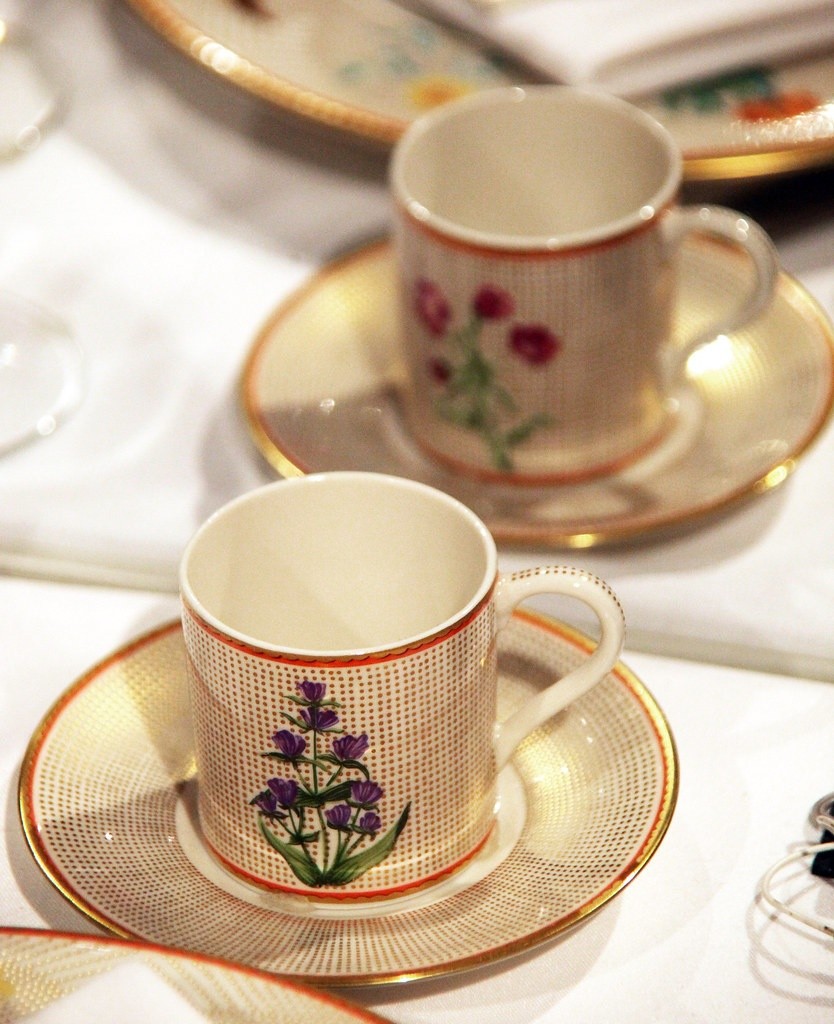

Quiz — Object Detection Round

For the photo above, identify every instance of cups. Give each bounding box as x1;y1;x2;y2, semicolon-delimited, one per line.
389;84;777;481
177;472;628;906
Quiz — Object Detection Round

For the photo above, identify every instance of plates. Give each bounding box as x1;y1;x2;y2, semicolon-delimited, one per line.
127;0;834;185
18;604;680;991
243;230;834;552
0;927;397;1024
